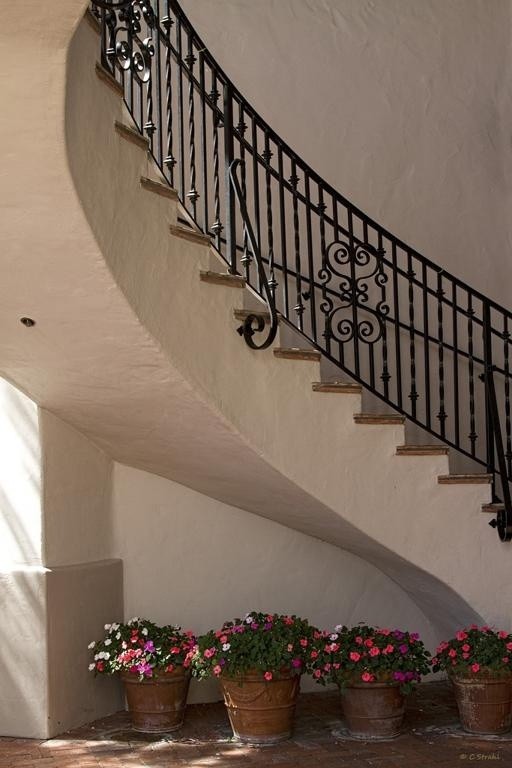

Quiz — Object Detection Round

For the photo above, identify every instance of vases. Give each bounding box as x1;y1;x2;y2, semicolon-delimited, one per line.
338;680;408;739
118;665;192;733
449;673;511;735
219;665;301;743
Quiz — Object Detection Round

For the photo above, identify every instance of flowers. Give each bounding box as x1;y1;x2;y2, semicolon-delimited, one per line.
85;610;196;685
429;621;512;686
197;611;323;685
323;616;432;685
